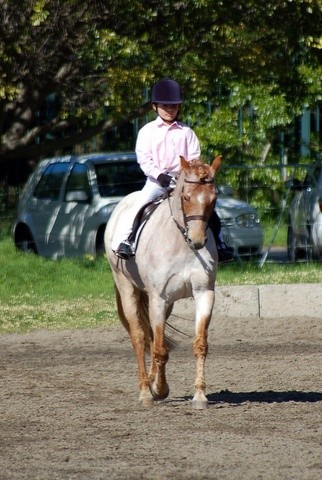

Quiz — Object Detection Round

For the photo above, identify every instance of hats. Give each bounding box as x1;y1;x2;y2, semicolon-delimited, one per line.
151;79;184;105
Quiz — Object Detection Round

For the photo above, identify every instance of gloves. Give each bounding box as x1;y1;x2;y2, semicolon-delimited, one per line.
157;173;172;188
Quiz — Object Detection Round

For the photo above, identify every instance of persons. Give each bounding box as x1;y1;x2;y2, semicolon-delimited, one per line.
117;80;234;262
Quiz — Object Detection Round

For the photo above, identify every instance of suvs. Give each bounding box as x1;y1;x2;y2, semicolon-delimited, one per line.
284;153;322;264
12;152;265;267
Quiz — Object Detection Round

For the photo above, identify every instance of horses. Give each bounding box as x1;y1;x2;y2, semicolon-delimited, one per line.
104;153;225;411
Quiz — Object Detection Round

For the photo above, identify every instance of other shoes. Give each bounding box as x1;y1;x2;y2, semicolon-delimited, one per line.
217;243;234;262
117;242;131;260
